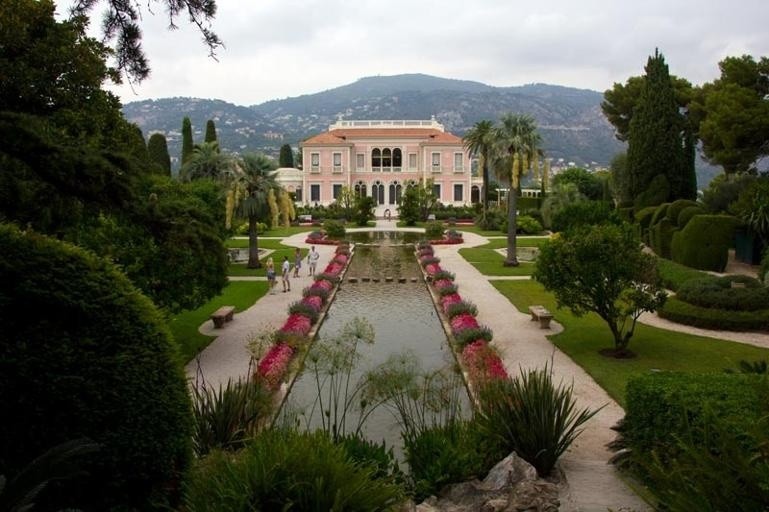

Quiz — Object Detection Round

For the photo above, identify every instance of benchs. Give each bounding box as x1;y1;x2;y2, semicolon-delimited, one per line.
529;306;554;329
209;306;236;328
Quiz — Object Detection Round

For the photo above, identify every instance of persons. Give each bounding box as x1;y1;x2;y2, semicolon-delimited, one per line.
293;248;302;278
307;246;319;276
266;256;275;294
281;256;291;292
